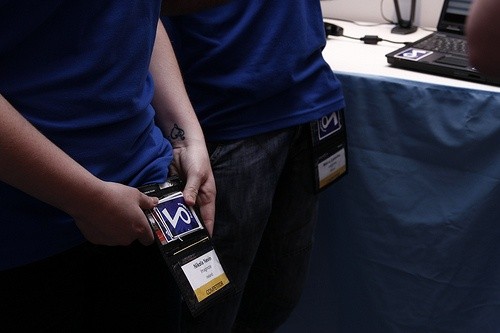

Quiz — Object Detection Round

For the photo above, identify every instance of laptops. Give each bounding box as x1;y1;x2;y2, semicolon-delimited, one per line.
384;0;482;83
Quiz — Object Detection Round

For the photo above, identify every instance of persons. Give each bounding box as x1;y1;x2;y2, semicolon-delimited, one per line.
0;0;217;333
160;0;349;333
464;0;500;82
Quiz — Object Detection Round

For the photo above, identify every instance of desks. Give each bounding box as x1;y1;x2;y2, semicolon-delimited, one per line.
275;18;500;333
